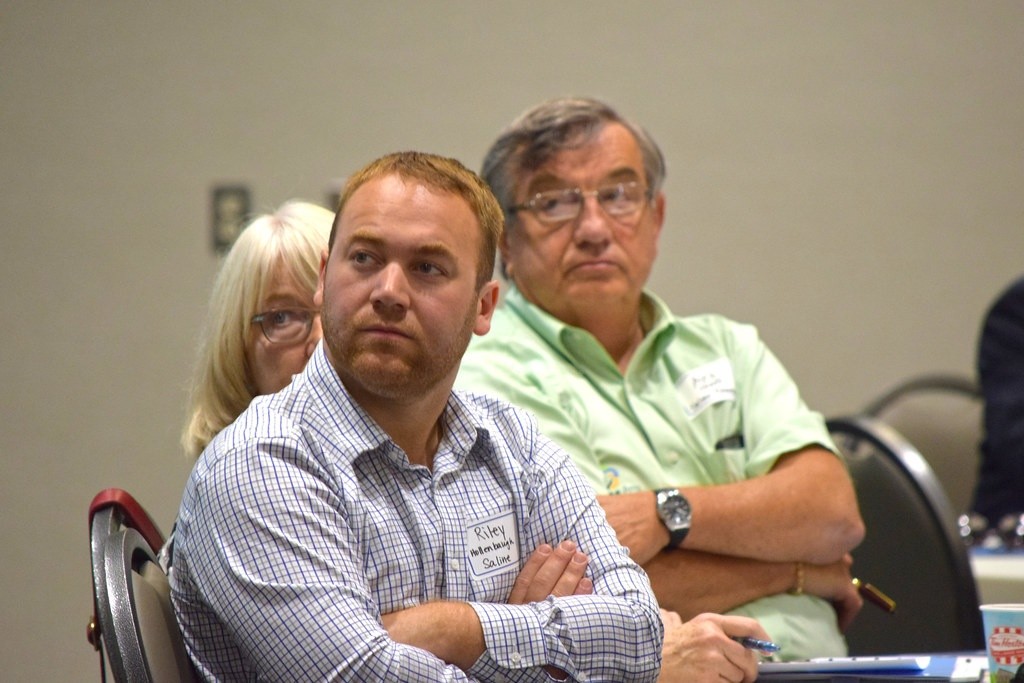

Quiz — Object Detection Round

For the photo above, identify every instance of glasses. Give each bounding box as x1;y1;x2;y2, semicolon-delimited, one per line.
247;305;320;345
507;180;651;221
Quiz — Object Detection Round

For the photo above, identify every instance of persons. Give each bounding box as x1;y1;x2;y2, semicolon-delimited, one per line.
967;274;1024;530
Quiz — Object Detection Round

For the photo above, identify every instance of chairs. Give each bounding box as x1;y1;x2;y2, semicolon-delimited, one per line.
90;488;196;683
825;370;988;658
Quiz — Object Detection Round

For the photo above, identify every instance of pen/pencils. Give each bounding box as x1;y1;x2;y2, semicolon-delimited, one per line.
729;634;782;654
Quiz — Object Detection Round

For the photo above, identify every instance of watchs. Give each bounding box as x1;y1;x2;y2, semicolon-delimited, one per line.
650;486;693;553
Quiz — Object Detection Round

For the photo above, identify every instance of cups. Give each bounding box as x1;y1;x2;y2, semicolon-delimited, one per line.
980;604;1024;682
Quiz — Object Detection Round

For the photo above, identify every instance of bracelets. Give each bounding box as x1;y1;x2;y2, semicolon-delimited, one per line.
787;561;805;596
154;96;896;683
